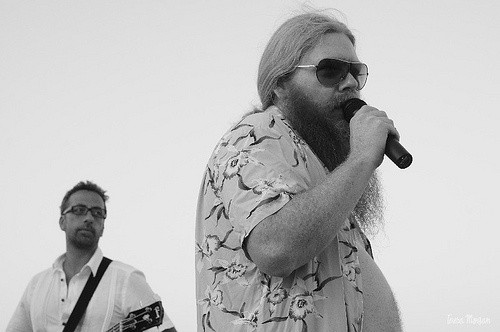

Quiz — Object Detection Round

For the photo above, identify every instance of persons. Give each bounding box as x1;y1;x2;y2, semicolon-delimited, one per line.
5;180;179;332
195;11;402;332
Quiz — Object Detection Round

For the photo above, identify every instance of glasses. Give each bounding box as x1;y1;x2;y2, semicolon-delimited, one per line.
295;57;370;92
62;204;106;220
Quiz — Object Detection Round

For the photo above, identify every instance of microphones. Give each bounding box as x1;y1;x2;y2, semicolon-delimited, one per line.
341;98;414;170
